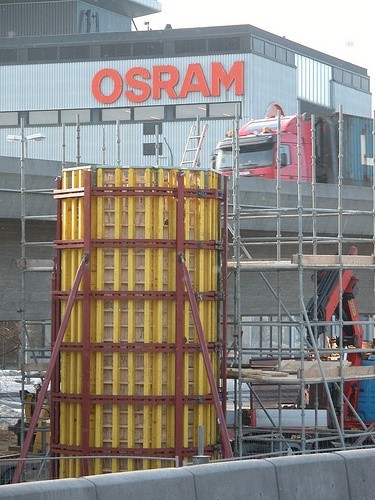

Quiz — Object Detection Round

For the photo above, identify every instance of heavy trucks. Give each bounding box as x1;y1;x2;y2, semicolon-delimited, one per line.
210;104;374;187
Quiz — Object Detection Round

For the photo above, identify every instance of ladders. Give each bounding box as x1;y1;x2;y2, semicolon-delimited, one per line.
177;125;207;169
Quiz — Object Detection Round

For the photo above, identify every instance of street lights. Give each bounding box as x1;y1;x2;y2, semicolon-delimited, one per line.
6;133;44;158
